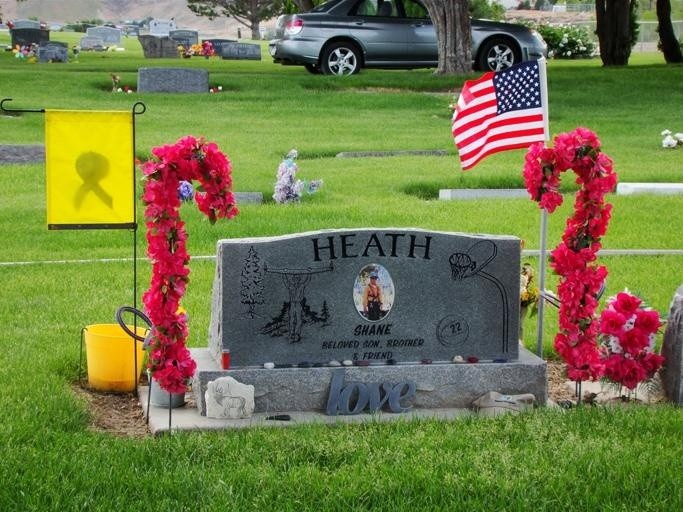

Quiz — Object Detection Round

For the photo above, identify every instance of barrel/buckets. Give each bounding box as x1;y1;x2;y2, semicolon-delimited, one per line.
84;324;149;392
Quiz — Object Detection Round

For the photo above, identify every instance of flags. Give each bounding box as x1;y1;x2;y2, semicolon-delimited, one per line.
451;60;546;172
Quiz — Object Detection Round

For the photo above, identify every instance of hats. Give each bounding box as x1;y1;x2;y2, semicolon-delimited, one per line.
368;272;379;278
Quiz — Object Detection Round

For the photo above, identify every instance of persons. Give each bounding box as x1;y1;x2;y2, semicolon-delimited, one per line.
363;271;383;320
283;267;312;343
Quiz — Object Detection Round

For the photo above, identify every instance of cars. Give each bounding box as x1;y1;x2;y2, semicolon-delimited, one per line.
269;0;548;77
